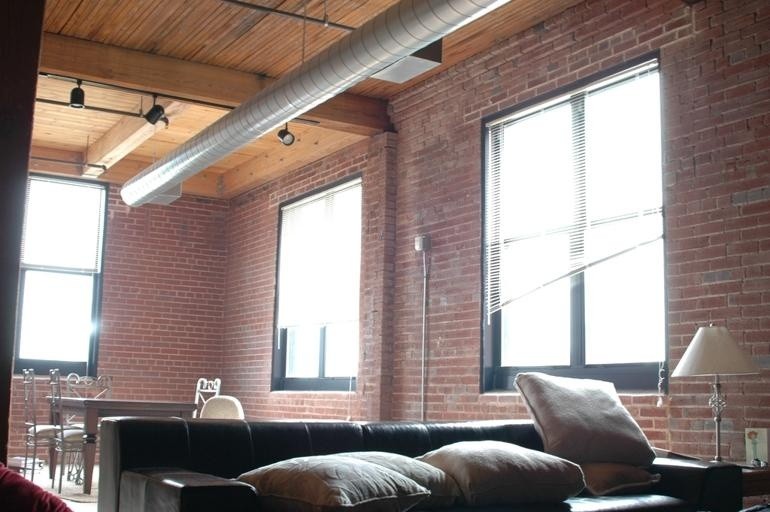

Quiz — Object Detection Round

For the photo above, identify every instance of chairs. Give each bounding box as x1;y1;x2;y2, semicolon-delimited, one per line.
21;366;221;499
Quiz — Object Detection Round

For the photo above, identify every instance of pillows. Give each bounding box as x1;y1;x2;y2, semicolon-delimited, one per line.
577;463;663;498
513;372;656;463
232;440;462;510
412;440;587;509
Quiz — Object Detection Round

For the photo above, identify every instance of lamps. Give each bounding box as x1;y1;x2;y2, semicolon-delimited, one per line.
276;120;295;146
69;79;86;108
669;320;762;464
142;92;170;128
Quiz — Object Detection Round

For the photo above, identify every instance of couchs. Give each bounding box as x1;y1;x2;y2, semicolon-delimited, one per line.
96;414;745;512
